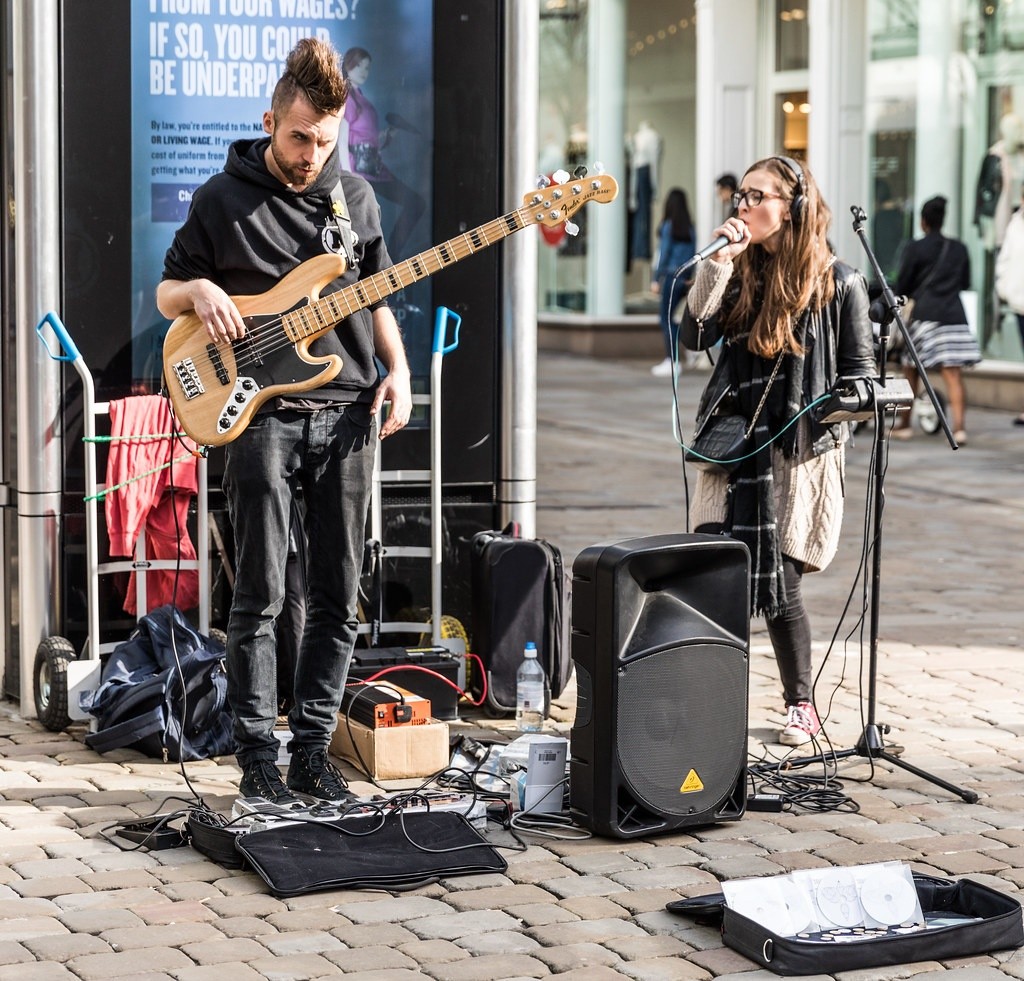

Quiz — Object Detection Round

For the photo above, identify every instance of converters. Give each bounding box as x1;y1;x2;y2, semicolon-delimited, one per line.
487;801;513;822
746;794;784;811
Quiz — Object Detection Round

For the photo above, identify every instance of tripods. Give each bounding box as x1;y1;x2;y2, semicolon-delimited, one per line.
745;205;978;803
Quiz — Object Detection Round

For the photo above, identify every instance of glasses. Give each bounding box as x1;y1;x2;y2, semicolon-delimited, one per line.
731;190;794;208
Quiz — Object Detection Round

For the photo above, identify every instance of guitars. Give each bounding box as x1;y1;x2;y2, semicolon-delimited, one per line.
160;159;620;449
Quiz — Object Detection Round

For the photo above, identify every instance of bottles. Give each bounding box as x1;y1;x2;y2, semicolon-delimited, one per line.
513;640;546;734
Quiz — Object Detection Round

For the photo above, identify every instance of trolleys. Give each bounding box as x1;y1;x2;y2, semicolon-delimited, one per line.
33;310;231;736
357;304;470;702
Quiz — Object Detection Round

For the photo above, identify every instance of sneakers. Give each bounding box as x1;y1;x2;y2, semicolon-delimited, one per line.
652;357;681;376
684;349;701;370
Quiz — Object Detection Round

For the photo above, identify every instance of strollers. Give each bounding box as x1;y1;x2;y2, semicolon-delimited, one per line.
848;298;947;435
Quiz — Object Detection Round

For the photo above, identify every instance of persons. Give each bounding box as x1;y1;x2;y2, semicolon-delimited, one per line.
650;173;1024;444
156;36;413;804
677;156;876;745
629;119;662;270
974;113;1024;333
338;47;424;264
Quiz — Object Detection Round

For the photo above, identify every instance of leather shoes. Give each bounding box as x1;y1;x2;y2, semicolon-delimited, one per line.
884;426;913;439
946;430;967;445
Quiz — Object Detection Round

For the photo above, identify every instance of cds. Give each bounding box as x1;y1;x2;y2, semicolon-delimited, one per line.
731;869;917;937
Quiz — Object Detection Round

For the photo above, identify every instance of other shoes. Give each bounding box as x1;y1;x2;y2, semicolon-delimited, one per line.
1014;413;1024;426
239;761;307;811
779;702;819;745
287;751;361;804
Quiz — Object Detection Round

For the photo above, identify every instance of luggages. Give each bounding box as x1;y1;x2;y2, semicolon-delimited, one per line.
470;523;572;718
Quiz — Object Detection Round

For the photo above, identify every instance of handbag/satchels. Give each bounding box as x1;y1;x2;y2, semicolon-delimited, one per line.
887;299;914;351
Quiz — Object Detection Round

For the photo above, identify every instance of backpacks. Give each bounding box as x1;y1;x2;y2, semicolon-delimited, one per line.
86;605;233;761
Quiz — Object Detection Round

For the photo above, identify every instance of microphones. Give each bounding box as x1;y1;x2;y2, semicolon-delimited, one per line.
679;229;744;270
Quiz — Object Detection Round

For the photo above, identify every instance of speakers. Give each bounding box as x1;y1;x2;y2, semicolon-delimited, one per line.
569;532;750;837
